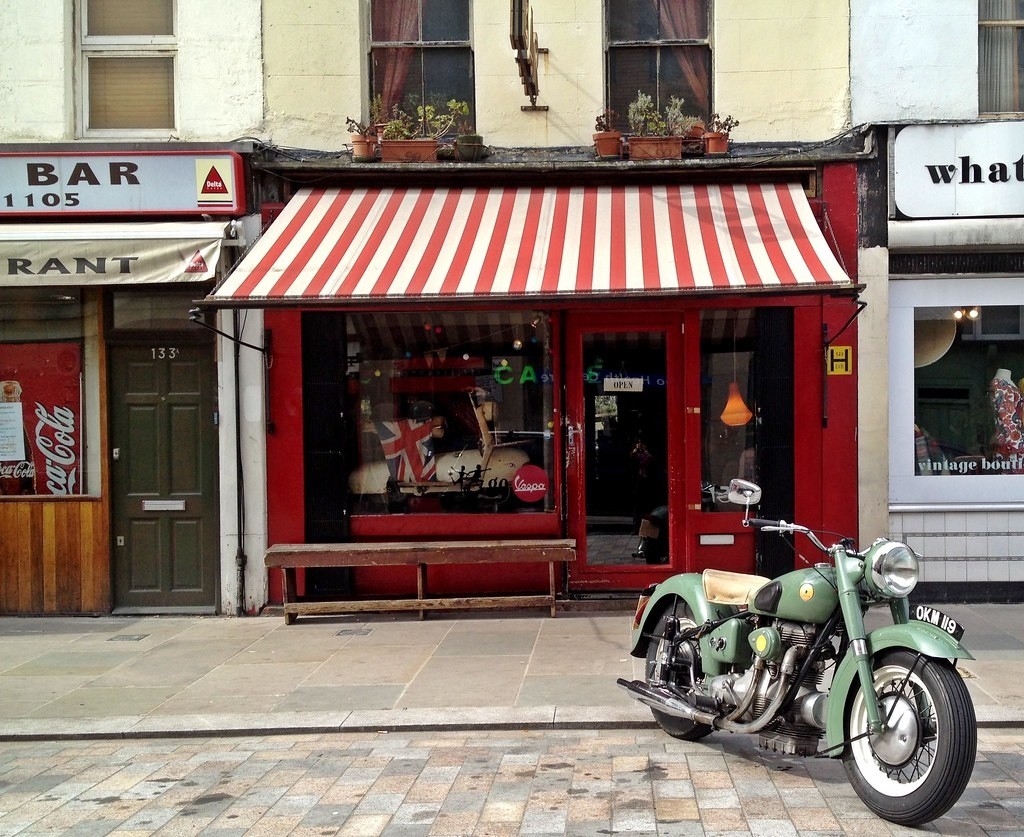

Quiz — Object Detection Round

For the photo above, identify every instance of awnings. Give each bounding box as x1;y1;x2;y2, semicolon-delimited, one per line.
187;188;870;348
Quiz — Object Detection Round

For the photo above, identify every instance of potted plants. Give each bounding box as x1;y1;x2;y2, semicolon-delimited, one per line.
703;113;740;154
379;99;469;163
368;93;406;139
593;108;622;155
345;116;378;157
628;89;702;160
675;115;705;137
458;120;483;162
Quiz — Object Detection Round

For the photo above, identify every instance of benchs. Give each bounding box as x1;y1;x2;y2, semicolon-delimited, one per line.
264;538;578;626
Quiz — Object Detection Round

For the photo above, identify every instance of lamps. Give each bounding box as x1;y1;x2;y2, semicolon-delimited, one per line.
720;319;753;427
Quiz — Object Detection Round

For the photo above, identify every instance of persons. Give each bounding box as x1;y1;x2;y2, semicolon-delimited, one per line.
627;435;652;536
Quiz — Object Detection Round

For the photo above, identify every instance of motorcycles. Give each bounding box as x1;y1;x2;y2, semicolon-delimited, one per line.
617;479;978;827
631;482;731;566
